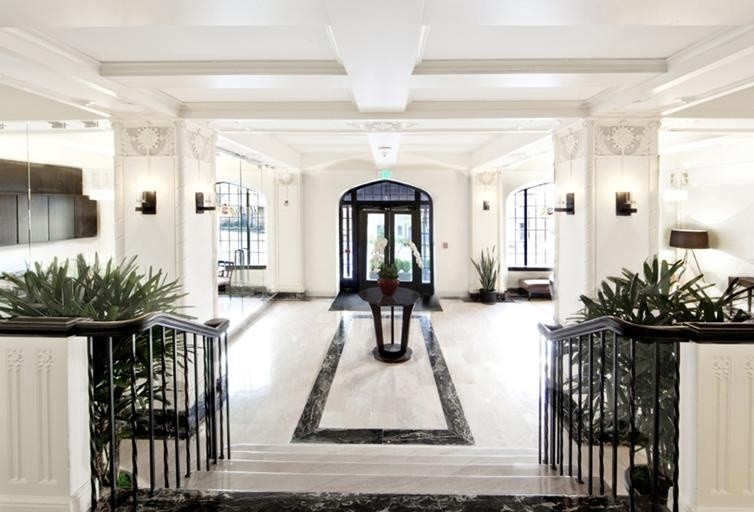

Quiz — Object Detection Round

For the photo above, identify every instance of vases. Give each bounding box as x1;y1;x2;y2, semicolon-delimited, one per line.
379;279;400;296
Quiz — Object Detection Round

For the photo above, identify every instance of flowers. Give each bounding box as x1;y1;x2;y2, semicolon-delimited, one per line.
370;235;424;278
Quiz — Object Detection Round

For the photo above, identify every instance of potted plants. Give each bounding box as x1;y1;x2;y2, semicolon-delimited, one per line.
470;245;497;305
552;253;754;502
0;250;204;492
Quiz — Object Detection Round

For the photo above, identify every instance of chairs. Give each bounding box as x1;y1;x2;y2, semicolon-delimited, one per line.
217;261;234;298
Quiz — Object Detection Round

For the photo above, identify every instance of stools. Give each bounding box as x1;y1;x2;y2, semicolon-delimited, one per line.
520;279;550;301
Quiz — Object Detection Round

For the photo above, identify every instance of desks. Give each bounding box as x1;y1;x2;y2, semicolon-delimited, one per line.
729;276;754;314
359;287;419;363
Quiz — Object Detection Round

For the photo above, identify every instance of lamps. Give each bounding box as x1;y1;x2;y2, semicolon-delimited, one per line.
134;191;156;215
615;188;636;216
669;229;709;282
195;192;214;214
554;192;575;215
